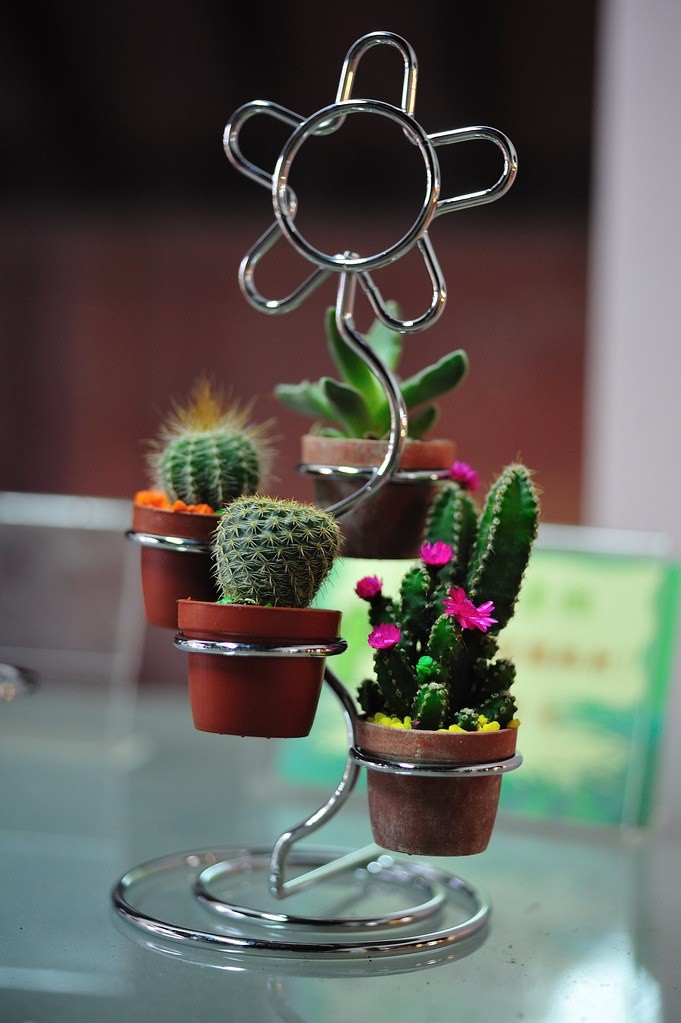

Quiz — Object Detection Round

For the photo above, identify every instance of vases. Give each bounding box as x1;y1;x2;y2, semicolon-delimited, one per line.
353;713;517;858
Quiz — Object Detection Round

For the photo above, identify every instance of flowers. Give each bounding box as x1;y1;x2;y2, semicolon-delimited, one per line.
354;461;542;733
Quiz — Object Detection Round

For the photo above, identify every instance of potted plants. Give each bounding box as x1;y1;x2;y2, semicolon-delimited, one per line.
273;300;468;560
177;492;342;738
133;377;285;629
